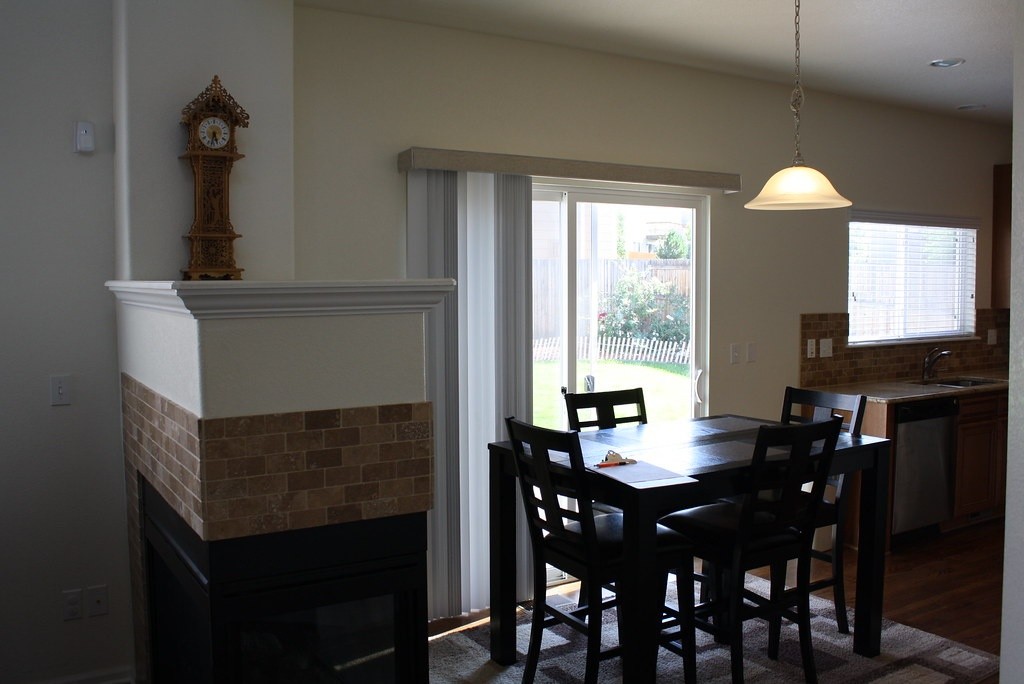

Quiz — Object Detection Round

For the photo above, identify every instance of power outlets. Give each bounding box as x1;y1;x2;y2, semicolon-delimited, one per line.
808;339;816;358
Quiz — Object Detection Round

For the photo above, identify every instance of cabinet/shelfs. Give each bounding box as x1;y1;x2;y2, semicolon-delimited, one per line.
953;418;1008;519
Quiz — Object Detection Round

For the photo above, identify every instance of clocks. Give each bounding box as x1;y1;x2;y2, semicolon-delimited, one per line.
175;76;250;283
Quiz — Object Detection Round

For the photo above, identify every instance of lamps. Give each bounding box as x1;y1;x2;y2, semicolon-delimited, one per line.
743;0;853;211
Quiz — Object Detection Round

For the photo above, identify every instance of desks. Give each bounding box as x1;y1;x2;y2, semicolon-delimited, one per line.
488;414;890;684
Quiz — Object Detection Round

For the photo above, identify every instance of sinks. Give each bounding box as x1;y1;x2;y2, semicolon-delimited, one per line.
938;376;998;387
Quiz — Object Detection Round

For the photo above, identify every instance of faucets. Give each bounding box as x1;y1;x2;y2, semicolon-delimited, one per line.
922;347;952;380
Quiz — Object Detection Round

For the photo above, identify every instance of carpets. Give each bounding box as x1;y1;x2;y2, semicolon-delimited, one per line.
272;572;1000;684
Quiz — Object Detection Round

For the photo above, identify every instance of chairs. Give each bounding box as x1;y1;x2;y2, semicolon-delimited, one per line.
657;413;844;684
700;385;867;659
505;415;697;684
564;388;707;648
